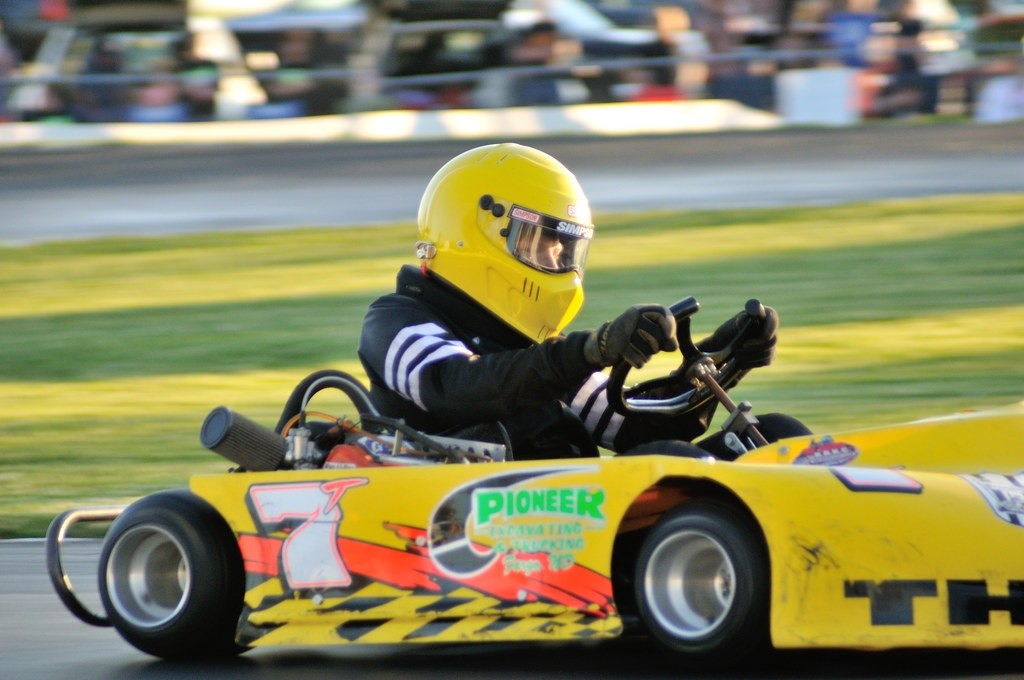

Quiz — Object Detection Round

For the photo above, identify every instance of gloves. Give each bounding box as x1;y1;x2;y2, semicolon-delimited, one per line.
583;302;679;372
700;305;779;370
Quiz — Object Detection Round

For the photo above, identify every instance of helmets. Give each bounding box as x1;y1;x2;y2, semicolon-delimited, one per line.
416;142;595;344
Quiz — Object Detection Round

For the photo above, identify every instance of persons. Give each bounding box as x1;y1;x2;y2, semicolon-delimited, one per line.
352;143;812;466
1;1;1024;128
